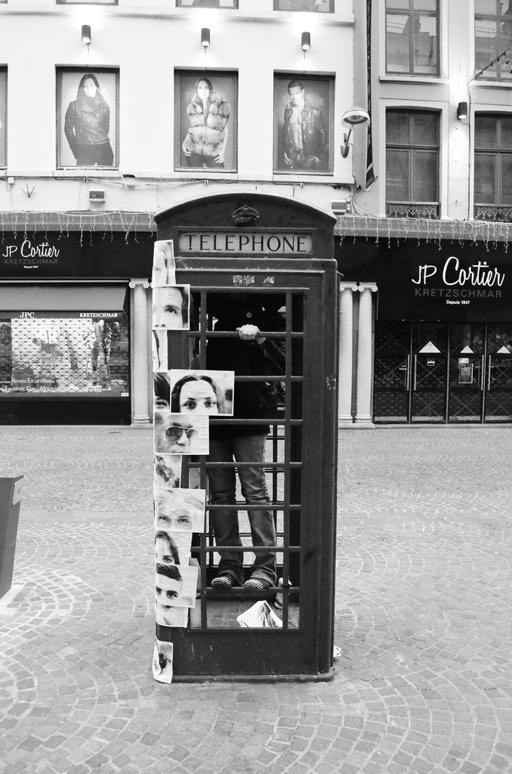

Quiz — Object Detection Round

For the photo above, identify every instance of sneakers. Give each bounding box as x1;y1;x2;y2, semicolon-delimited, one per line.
211;574;235;588
244;579;271;590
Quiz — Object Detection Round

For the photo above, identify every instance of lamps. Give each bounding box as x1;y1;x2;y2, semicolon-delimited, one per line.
457;102;467;119
340;106;371;158
82;25;92;56
201;28;210;55
301;32;310;63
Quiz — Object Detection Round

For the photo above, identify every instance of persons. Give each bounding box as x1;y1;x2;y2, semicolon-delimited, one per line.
153;241;224;606
283;81;326;170
197;290;287;592
181;78;231;167
64;73;113;166
